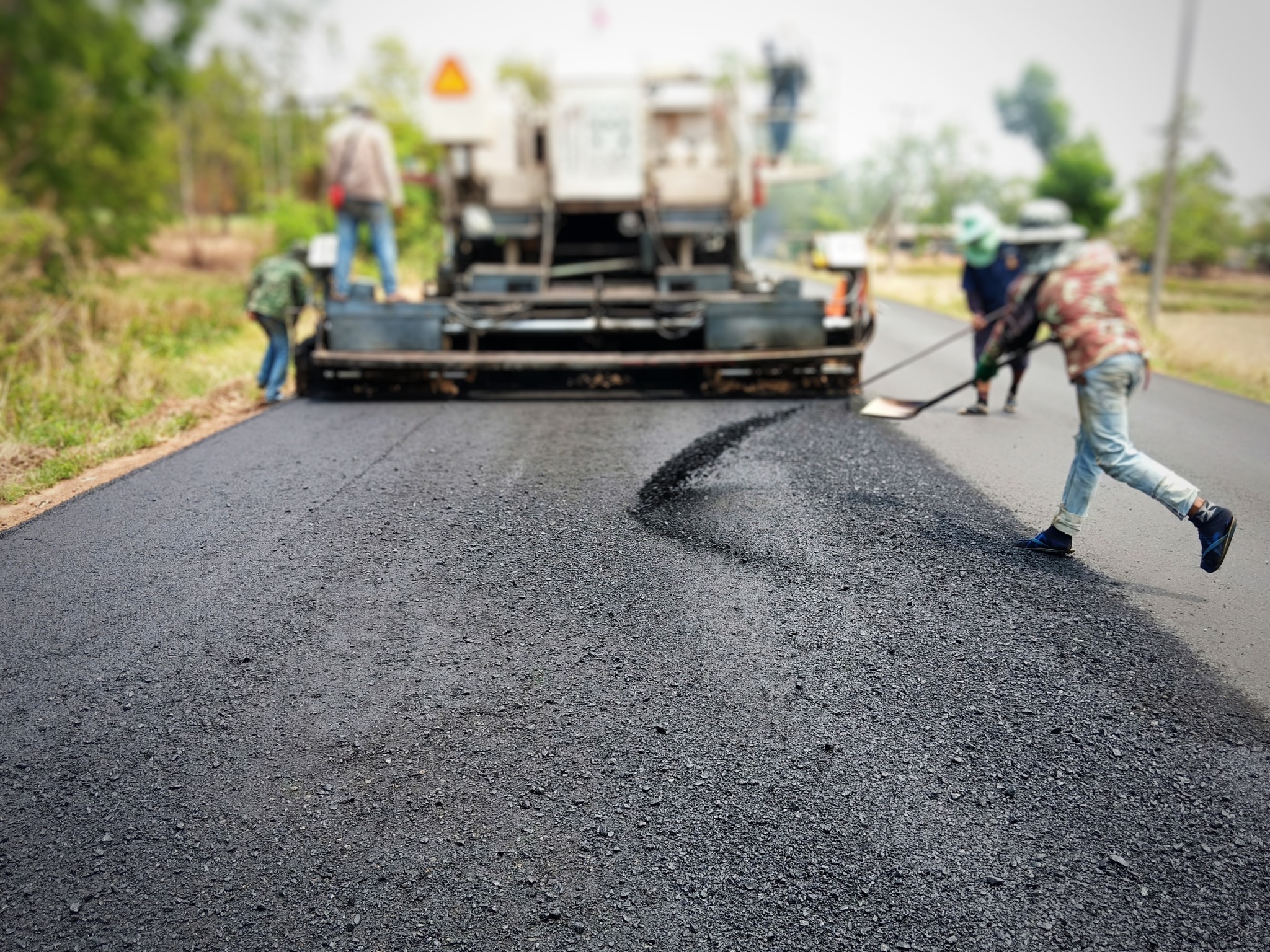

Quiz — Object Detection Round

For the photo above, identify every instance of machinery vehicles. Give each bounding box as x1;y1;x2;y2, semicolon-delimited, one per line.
295;64;876;403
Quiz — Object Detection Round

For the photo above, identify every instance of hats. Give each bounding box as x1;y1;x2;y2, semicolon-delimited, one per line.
997;199;1085;244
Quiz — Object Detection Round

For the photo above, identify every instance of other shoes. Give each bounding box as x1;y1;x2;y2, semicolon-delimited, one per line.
959;401;988;415
331;291;348;303
385;293;409;305
259;395;283;406
1003;393;1019;414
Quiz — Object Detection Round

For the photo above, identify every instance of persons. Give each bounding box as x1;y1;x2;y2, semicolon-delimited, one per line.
247;225;337;406
325;99;413;304
954;203;1034;412
758;39;811;170
975;199;1238;574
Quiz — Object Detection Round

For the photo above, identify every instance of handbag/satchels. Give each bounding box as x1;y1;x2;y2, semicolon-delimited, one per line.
329;180;345;209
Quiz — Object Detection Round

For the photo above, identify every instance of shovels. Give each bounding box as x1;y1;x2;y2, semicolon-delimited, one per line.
857;334;1064;419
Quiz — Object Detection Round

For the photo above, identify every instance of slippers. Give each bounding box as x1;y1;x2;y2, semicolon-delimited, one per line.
1200;516;1237;573
1016;532;1076;554
